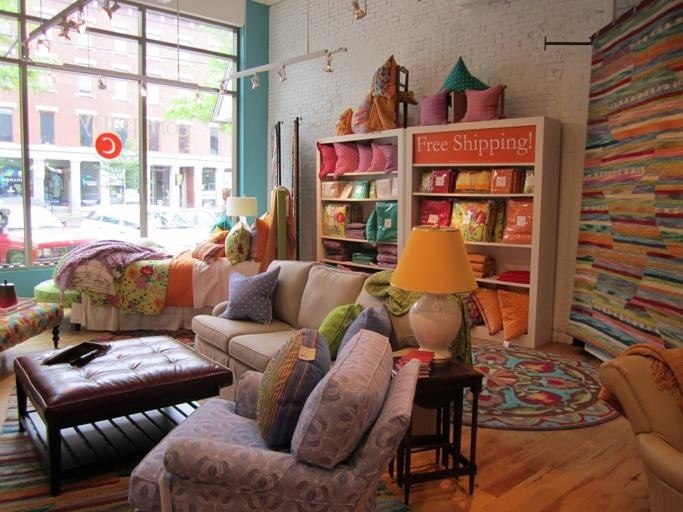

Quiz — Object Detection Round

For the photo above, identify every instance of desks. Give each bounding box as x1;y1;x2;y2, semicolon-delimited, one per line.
384;355;483;506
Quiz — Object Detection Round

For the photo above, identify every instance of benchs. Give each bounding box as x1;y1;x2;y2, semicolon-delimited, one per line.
13;333;233;498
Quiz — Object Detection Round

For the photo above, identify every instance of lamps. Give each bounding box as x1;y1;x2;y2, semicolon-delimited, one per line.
93;0;204;103
217;0;347;95
18;1;121;63
350;0;366;19
225;196;257;226
388;223;479;368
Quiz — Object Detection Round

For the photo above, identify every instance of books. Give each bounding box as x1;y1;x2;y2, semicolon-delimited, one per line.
394;349;435;379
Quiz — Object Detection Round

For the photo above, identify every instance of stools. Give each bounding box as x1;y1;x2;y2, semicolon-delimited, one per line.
0;297;63;354
34;277;81;308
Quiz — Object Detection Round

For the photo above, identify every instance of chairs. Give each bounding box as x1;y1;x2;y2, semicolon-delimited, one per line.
128;358;420;512
598;347;683;512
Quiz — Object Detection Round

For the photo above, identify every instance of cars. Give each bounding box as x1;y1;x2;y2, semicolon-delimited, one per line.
0;199;89;265
88;204;220;245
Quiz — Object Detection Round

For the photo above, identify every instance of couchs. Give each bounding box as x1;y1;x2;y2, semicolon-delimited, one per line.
190;260;467;435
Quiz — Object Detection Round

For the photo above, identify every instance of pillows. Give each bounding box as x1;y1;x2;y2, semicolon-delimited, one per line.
459;285;528;342
216;265;281;327
335;54;509;138
256;300;396;465
316;141;398;179
191;208;269;265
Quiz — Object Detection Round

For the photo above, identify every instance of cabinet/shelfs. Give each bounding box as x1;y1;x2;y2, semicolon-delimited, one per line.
315;126;401;273
404;116;559;351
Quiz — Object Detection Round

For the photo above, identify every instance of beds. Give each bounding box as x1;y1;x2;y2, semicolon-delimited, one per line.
67;185;291;331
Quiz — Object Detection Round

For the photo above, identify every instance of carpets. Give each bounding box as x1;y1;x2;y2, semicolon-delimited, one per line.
0;383;130;512
88;329;195;348
439;342;622;431
